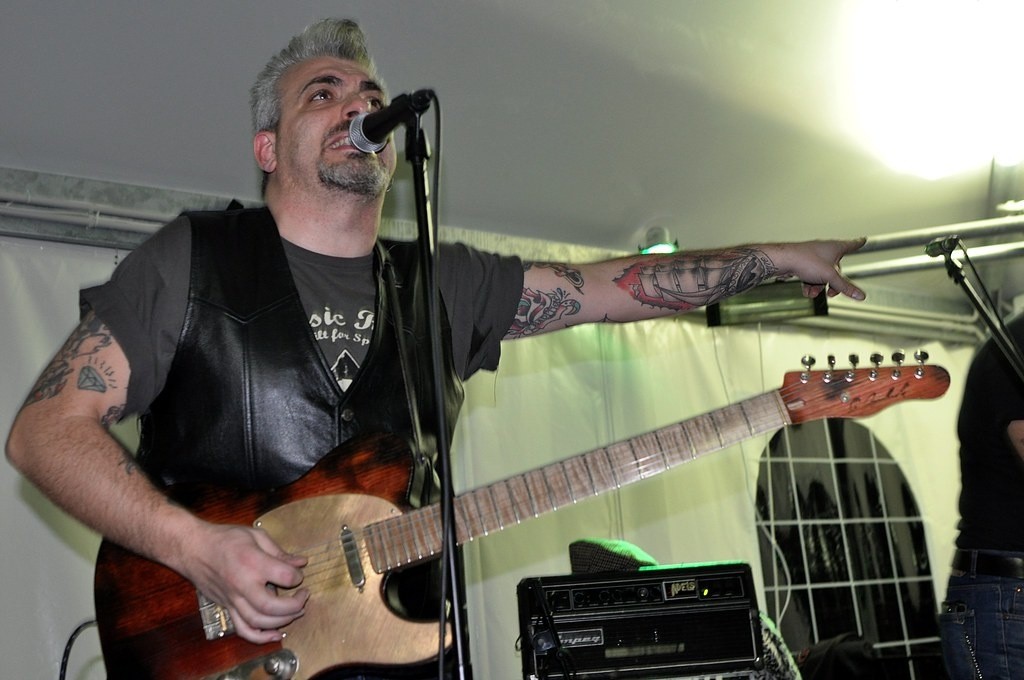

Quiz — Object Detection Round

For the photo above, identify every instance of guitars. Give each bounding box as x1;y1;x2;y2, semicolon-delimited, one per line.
91;350;954;680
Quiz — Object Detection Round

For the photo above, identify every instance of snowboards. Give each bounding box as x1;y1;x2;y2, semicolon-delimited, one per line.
752;417;943;680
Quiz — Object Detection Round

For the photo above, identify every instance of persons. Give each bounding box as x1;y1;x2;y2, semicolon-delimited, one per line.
4;20;867;680
940;310;1024;680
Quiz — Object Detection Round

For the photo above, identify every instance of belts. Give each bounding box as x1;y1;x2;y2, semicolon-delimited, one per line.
952;551;1024;580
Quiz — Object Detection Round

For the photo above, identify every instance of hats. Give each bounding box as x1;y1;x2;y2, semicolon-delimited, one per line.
569;537;658;573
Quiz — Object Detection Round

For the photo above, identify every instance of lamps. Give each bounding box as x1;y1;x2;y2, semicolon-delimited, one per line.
639;228;679;253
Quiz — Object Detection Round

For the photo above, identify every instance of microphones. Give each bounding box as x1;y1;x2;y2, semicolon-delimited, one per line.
348;90;433;153
924;236;959;257
